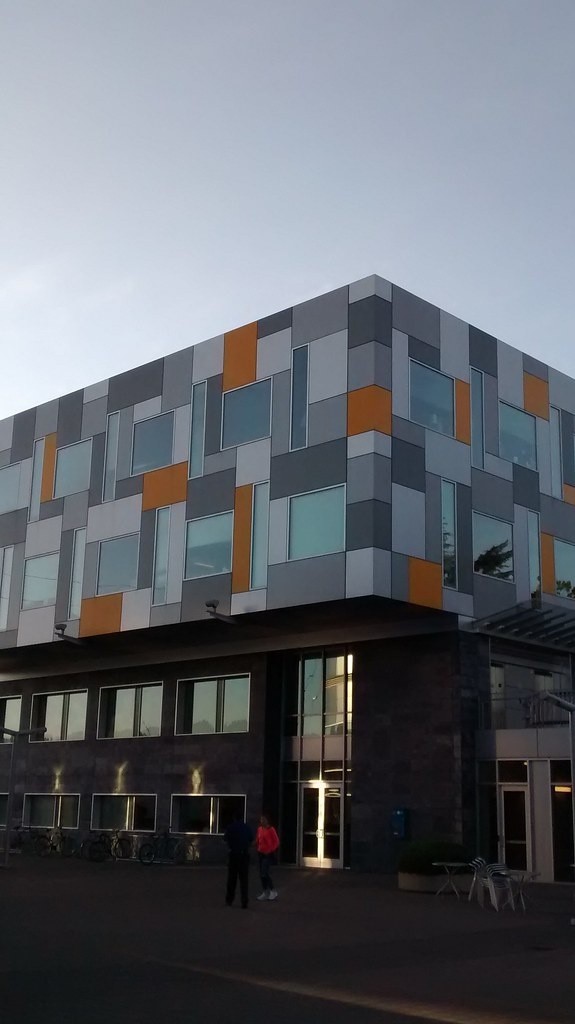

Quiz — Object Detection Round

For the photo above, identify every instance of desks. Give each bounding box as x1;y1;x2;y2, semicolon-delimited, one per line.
501;870;541;914
432;861;467;901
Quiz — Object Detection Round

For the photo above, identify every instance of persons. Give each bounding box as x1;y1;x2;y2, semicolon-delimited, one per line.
256;816;280;901
223;812;252;908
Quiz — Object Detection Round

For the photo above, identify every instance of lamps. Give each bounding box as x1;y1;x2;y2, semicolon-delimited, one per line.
205;599;246;626
55;623;86;647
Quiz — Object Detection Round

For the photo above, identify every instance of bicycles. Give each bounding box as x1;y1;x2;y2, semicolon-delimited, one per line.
138;826;196;866
7;825;133;860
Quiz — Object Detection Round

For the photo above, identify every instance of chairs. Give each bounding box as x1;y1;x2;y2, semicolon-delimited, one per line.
468;856;516;914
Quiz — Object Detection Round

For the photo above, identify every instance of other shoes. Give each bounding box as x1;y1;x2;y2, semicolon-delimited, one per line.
256;891;278;900
